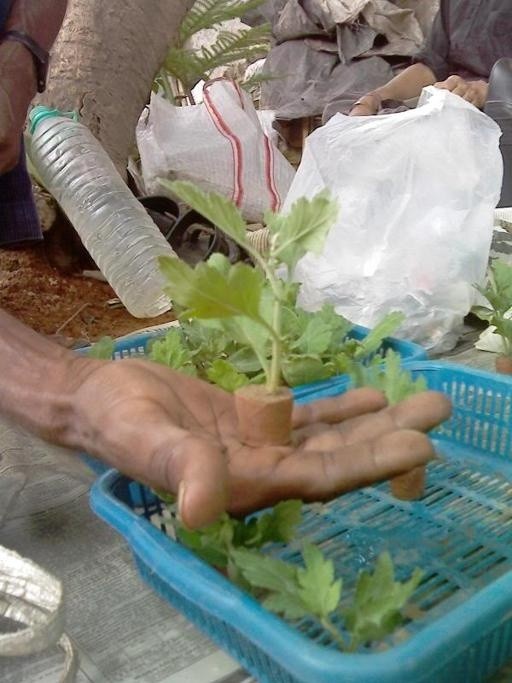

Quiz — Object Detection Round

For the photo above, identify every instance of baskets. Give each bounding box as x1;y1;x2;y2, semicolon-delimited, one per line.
74;322;428;508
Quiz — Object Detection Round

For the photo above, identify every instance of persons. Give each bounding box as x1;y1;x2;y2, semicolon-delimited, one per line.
0;0;70;254
0;289;454;536
346;1;512;208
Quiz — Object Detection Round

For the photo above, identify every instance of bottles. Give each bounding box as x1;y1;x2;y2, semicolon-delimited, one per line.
22;103;181;319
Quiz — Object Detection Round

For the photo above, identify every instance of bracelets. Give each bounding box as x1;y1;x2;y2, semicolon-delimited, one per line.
0;28;52;100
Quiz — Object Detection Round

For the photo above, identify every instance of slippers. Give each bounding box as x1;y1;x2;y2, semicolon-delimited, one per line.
136;195;181;236
165;208;242;269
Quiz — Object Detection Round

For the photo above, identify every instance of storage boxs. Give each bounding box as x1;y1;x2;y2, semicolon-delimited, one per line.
75;306;429;474
